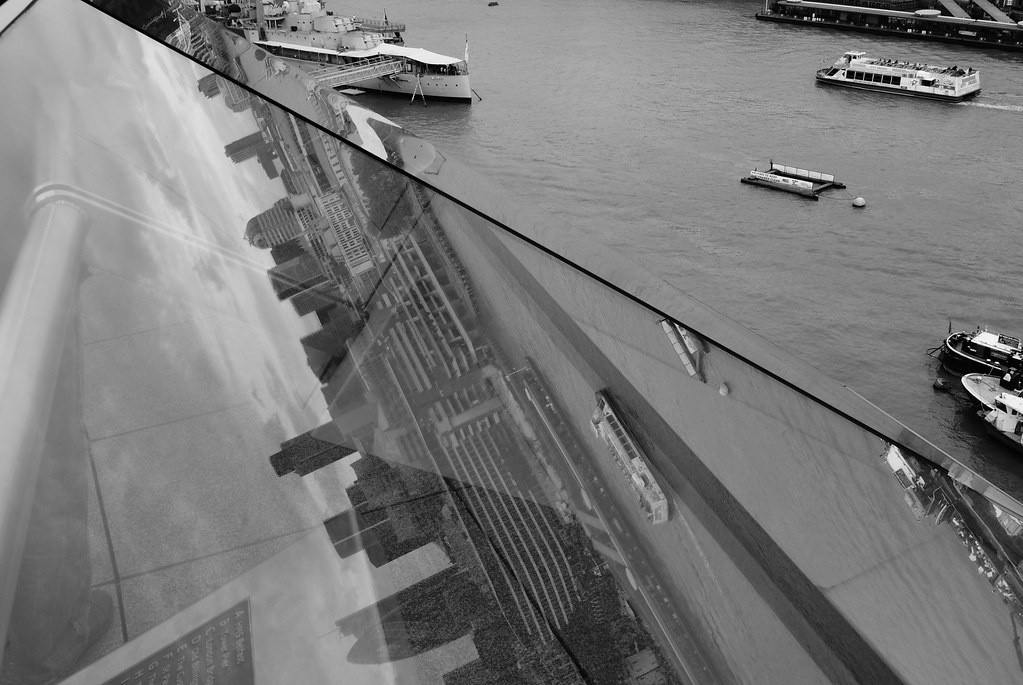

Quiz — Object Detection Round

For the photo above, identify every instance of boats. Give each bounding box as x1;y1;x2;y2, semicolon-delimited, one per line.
887;444;1023;627
177;0;473;105
961;368;1023;452
816;51;982;103
926;319;1023;378
590;390;673;524
